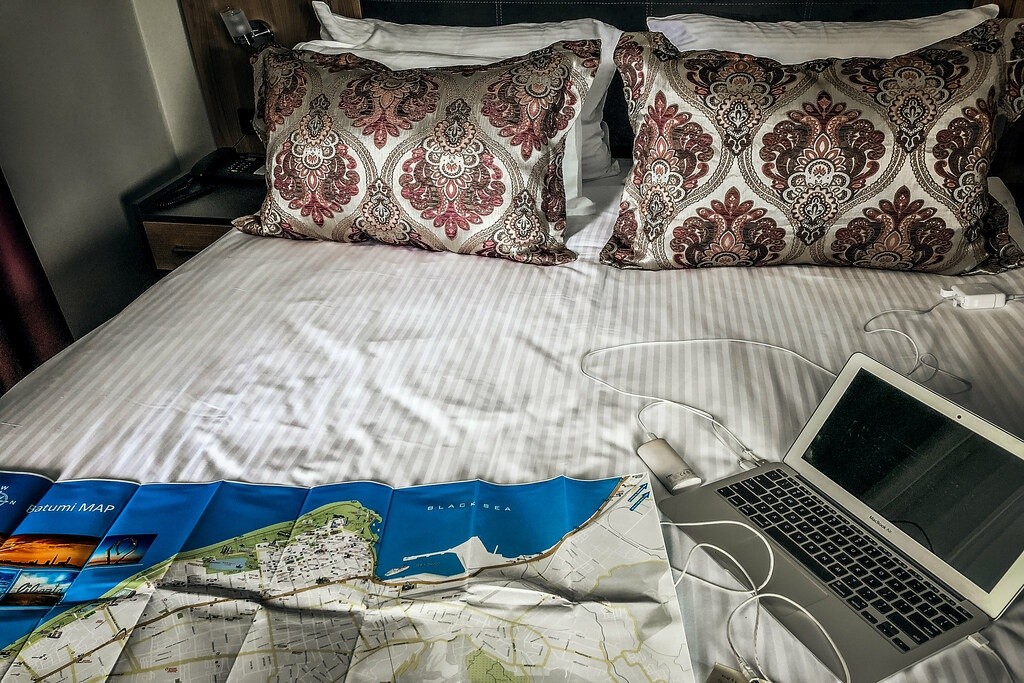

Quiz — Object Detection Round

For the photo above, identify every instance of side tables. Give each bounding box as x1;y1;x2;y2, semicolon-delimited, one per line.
132;164;269;280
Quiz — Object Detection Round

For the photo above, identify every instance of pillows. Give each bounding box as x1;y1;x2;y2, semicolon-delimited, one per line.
292;40;598;215
311;1;626;181
598;17;1024;277
228;40;602;266
646;3;1001;64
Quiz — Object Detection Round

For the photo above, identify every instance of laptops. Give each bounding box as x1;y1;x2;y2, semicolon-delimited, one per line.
657;351;1024;683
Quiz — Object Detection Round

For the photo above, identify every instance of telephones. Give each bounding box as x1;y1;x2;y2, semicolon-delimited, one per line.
190;147;266;183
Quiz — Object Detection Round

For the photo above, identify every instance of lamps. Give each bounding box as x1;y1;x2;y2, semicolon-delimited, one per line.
219;7;272;55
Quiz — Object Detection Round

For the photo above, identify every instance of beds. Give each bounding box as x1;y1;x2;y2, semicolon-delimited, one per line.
0;158;1024;683
